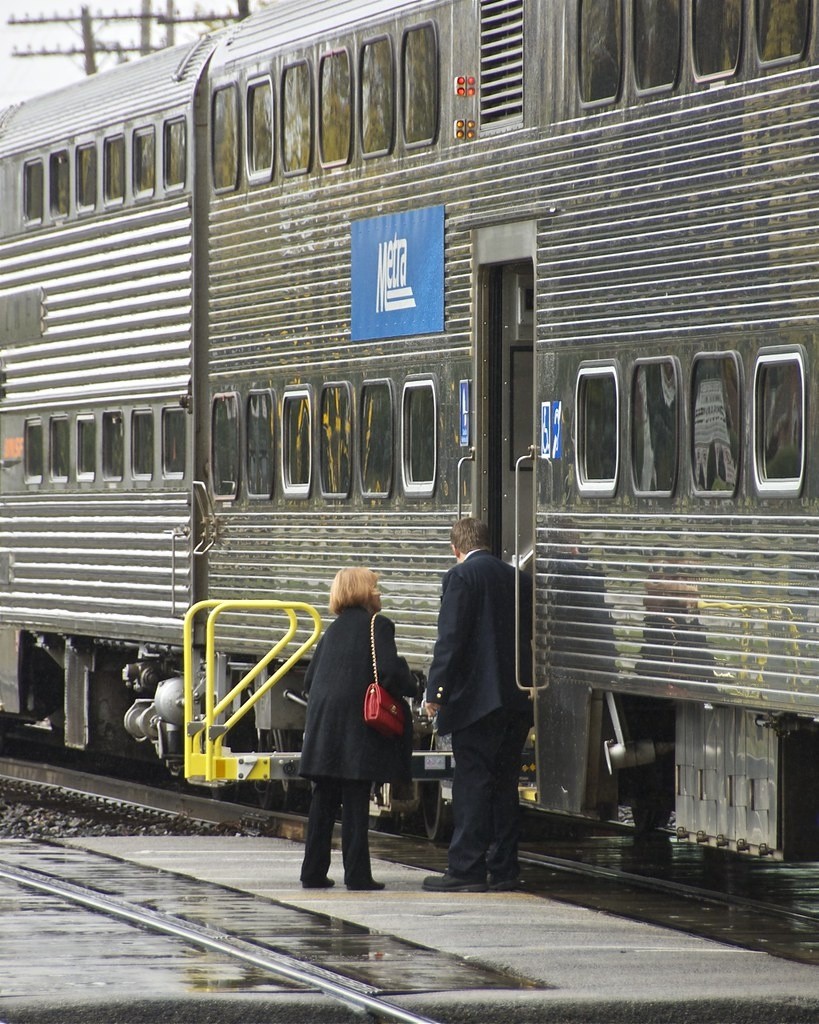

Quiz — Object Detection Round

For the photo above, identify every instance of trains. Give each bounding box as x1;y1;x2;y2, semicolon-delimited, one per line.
0;0;819;864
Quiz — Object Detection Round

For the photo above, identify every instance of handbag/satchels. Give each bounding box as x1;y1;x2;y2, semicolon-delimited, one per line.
363;611;404;737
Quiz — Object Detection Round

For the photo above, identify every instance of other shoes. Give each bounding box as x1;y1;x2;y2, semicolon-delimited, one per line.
346;878;384;890
302;876;334;888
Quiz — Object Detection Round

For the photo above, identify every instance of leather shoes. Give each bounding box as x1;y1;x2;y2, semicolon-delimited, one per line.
485;873;516;890
422;872;489;893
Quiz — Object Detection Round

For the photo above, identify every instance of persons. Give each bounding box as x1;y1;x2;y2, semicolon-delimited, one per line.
422;517;533;891
297;568;427;891
